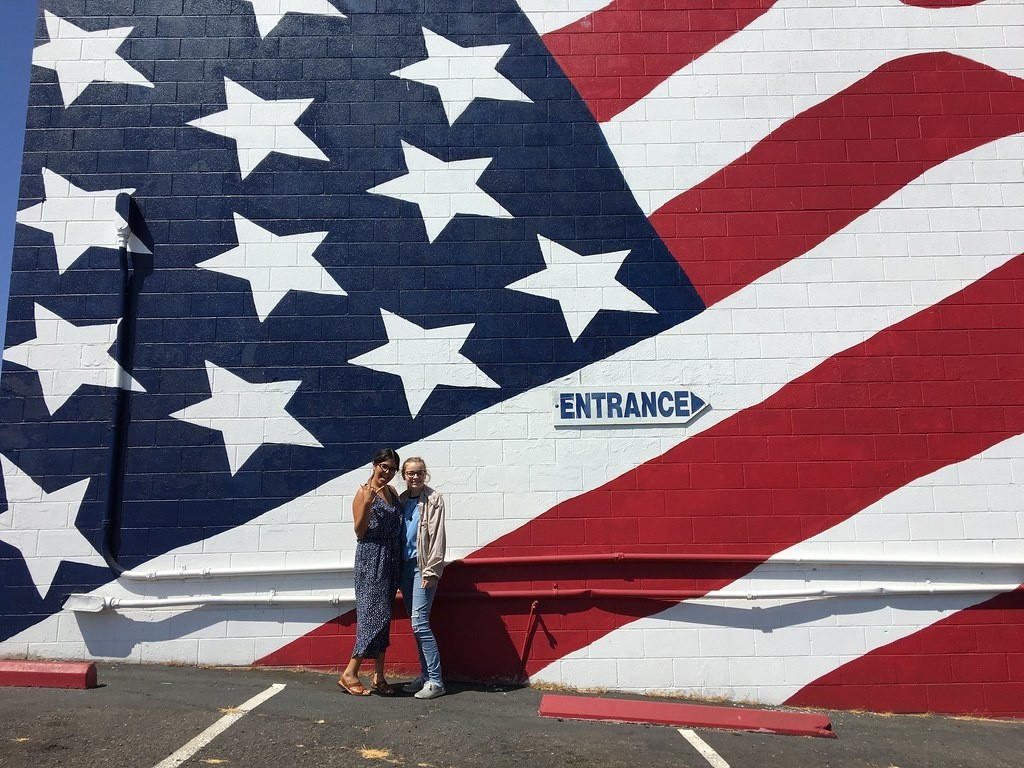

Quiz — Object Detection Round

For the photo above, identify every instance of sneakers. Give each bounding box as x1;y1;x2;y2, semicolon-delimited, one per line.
414;681;445;699
402;677;426;691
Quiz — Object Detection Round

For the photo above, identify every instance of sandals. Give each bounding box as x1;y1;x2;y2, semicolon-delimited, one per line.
370;679;395;695
338;675;371;696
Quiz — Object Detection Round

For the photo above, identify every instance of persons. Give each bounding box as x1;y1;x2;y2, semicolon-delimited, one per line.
398;456;448;700
337;449;403;697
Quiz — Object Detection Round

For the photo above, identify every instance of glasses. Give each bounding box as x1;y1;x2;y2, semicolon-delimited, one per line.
405;470;426;478
377;464;398;475
